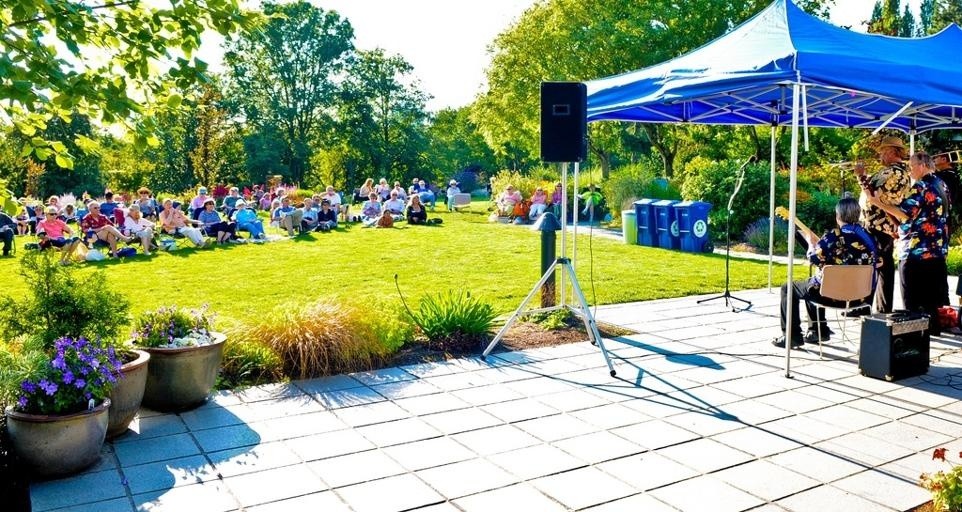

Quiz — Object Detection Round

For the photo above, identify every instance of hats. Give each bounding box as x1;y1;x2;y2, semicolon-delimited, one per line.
875;135;908;154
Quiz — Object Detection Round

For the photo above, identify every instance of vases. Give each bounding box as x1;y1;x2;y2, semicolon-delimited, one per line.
5;396;111;478
125;332;228;414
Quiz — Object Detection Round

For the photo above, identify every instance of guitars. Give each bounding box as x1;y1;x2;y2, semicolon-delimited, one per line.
860;176;907;227
775;205;821;244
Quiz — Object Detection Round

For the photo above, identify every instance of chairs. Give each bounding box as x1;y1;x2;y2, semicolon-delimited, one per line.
581;188;603;221
497;189;562;225
270;190;435;237
441;188;471;220
0;188;264;264
808;263;875;360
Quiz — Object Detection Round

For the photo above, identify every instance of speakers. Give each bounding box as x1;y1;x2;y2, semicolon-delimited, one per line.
540;81;587;160
857;309;931;382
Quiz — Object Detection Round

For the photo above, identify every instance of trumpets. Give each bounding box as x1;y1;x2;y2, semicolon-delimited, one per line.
830;158;884;172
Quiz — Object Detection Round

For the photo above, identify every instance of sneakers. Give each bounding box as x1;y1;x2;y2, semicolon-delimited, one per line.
125;237;137;245
803;330;831;343
772;335;805;348
59;258;74;266
197;237;212;250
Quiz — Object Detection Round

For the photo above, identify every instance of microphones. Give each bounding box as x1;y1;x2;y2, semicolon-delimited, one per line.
742;155;756;168
839;160;844;179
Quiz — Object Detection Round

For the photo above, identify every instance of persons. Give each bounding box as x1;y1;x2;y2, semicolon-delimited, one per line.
864;151;951;338
838;135;909;318
930;147;961;232
770;196;878;348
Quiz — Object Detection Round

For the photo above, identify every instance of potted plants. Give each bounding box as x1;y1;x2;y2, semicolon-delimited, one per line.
1;242;151;442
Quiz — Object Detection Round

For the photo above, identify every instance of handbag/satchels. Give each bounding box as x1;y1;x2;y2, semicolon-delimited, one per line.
86;239;178;262
427;217;442;226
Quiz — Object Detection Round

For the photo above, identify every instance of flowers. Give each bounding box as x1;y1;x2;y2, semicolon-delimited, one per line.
130;305;222;347
12;332;127;415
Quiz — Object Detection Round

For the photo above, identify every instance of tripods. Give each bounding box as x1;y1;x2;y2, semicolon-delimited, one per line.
696;167;751;312
481;163;615;376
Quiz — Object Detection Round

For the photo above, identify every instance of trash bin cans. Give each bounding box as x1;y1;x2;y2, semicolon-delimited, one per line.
621;198;713;253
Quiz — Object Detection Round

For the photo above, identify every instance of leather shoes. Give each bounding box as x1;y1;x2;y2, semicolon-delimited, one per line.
841;306;871;317
